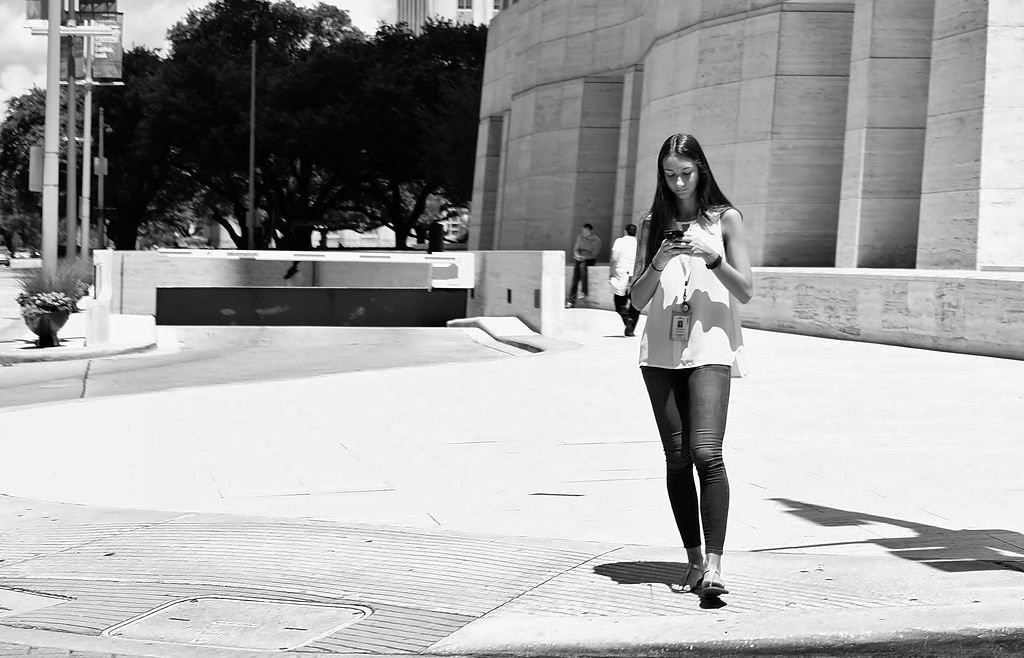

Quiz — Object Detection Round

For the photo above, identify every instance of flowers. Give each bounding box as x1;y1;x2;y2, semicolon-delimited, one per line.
9;254;94;327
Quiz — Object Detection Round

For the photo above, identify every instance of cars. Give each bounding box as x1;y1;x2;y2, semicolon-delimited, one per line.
0;246;11;266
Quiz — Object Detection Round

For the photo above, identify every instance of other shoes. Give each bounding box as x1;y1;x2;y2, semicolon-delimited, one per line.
565;302;574;308
578;292;587;299
625;331;634;336
625;318;634;331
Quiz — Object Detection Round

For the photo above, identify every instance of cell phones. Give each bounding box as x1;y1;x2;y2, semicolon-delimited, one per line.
664;229;684;243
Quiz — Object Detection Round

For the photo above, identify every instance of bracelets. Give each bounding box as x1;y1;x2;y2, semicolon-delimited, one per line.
705;252;723;270
650;260;664;272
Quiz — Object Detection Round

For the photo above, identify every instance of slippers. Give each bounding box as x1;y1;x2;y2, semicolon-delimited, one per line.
671;562;703;593
702;569;729;597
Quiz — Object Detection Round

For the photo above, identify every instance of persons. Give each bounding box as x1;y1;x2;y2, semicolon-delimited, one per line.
565;223;602;308
628;133;754;598
608;224;641;337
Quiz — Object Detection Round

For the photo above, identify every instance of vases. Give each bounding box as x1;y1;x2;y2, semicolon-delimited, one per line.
23;301;84;347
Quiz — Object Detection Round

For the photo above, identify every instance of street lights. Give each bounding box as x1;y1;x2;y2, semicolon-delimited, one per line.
97;106;114;250
